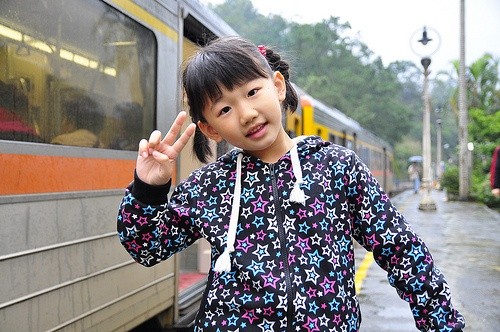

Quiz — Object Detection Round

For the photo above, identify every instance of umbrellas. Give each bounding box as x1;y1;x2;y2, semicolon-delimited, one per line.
408;156;423;163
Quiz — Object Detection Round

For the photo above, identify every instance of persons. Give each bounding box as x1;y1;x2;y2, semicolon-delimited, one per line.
115;36;467;332
0;77;144;152
489;146;500;196
407;162;420;195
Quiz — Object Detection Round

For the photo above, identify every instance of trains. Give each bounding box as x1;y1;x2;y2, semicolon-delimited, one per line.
0;1;395;332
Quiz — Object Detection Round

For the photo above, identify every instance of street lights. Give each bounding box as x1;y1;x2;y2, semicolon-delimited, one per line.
434;107;444;191
418;59;436;211
